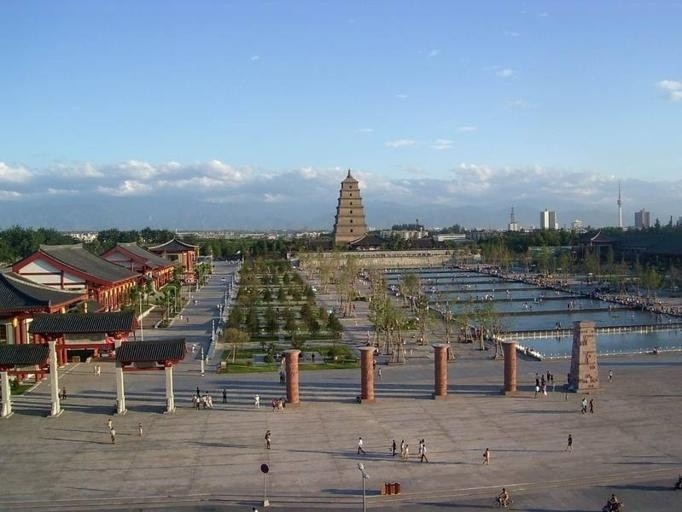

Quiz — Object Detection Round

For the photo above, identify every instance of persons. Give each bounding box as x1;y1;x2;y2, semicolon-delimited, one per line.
499;487;509;507
265;430;271;449
564;434;573;453
357;437;367;454
139;423;143;436
609;493;617;511
105;419;112;433
392;439;429;464
110;427;116;444
483;448;489;465
676;474;682;488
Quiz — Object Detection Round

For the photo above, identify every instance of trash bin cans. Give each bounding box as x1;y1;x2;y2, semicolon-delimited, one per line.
385;482;400;495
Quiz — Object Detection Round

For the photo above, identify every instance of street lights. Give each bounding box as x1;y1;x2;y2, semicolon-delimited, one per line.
139;291;143;341
357;462;370;511
